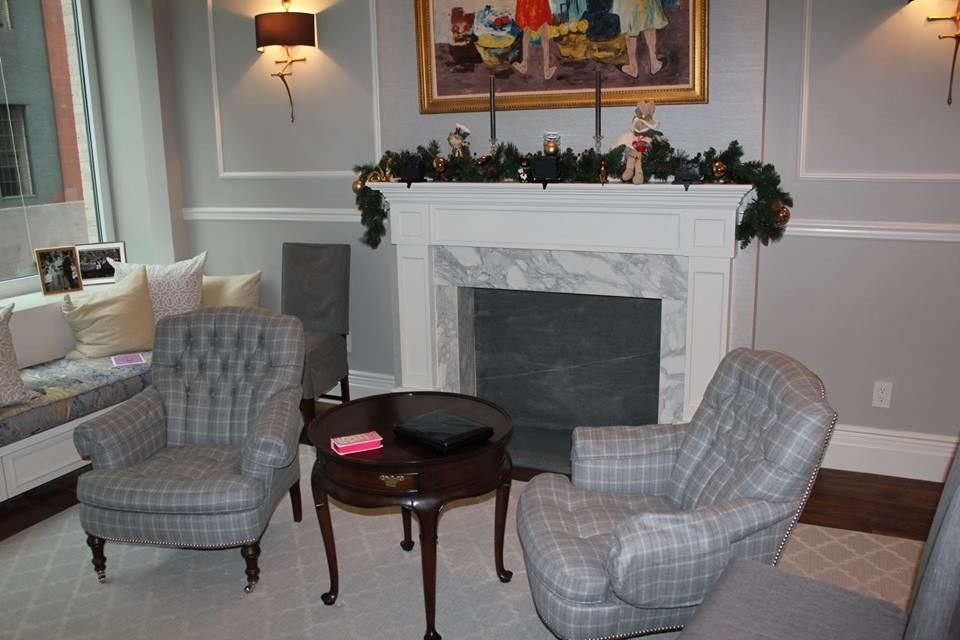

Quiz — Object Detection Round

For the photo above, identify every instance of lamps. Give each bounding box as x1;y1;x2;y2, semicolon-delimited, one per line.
255;4;315;124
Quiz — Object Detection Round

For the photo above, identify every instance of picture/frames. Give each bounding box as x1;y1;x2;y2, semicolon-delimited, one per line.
73;241;128;287
415;1;709;115
34;245;84;295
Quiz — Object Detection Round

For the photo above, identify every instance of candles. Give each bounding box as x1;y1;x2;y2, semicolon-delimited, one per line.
596;70;601;136
489;75;496;139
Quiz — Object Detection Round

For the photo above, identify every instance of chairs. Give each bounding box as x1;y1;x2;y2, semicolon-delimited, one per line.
679;434;959;640
277;242;350;437
72;307;306;593
515;347;838;640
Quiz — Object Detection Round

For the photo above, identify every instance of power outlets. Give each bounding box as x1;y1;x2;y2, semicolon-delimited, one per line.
871;380;892;409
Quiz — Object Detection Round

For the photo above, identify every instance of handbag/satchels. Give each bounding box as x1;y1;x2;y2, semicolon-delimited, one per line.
393;409;495;457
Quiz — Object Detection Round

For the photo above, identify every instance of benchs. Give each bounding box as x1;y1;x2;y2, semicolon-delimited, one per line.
0;273;262;503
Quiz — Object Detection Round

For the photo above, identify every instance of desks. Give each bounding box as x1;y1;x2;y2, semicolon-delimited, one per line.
307;391;515;637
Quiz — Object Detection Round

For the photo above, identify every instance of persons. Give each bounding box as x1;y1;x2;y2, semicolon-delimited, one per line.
50;251;75;289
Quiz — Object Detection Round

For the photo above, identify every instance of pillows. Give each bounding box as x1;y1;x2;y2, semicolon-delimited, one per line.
0;302;43;409
107;252;206;348
194;269;263;307
62;265;156;360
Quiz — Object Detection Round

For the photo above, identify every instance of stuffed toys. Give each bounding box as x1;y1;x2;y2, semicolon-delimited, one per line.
621;100;660;184
